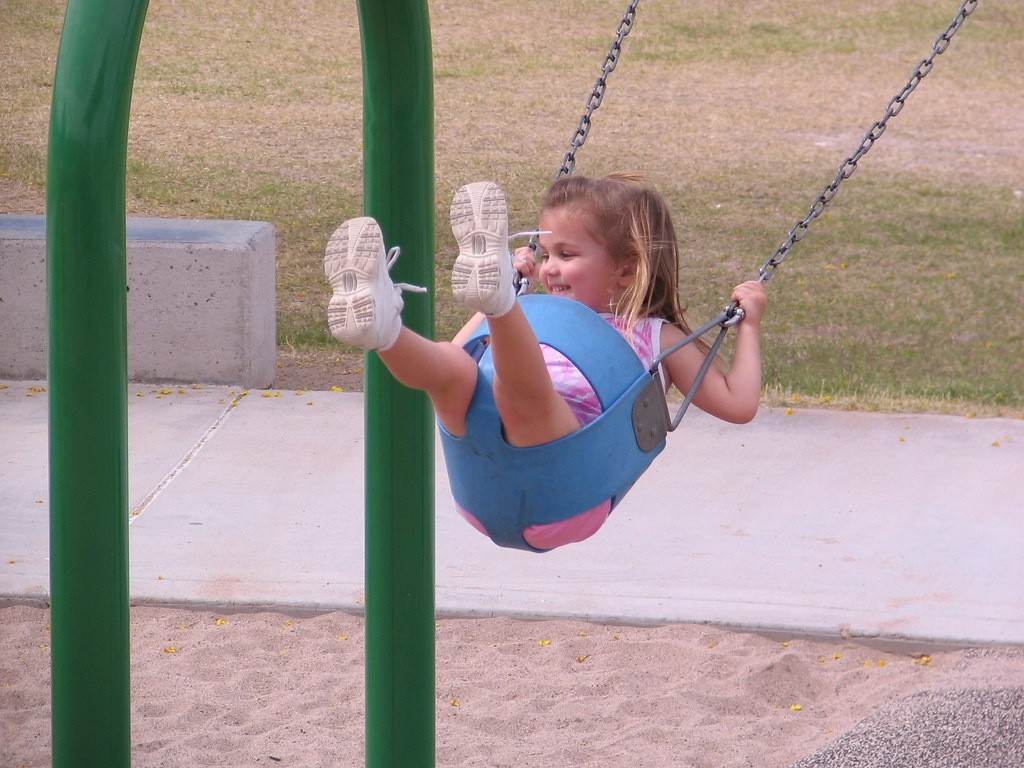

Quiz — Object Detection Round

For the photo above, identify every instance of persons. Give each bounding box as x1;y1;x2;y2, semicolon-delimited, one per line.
322;175;765;551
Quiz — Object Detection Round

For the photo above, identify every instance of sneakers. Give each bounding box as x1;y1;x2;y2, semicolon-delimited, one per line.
324;216;428;351
450;181;552;315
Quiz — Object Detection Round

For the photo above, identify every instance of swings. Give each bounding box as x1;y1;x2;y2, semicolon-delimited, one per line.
437;1;981;554
